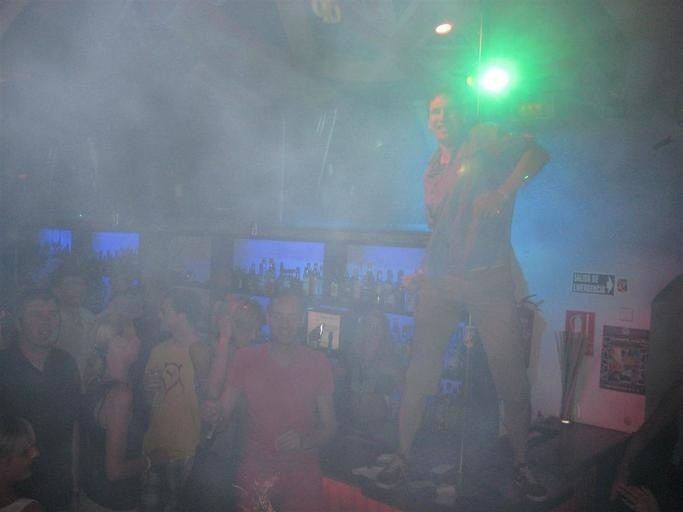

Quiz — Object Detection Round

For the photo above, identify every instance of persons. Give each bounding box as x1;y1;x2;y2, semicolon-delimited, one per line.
370;88;550;503
1;254;683;512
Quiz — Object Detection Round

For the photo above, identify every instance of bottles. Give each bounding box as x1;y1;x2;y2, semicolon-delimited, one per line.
249;257;417;312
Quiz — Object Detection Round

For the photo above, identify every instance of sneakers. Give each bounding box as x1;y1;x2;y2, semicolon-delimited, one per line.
376;459;410;492
509;458;546;502
